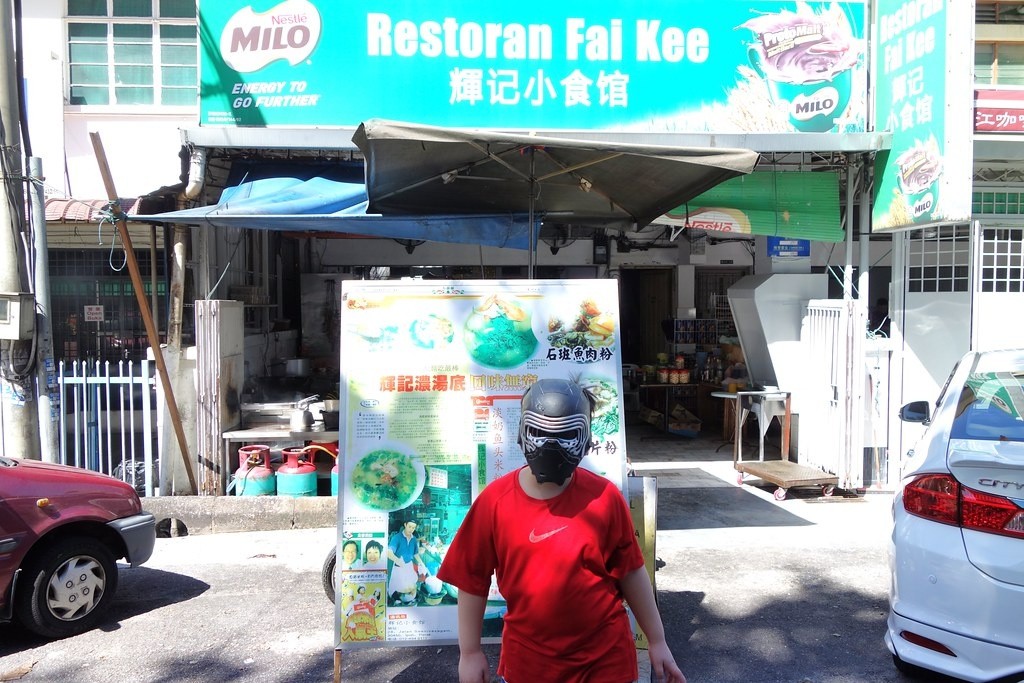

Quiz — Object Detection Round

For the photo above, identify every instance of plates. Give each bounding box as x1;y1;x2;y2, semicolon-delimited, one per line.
531;291;613;351
462;301;539;371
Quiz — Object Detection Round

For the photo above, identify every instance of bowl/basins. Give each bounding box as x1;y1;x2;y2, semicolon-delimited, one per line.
420;583;447;605
345;440;425;512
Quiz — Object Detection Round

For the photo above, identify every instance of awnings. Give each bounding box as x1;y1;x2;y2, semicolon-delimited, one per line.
124;177;537;250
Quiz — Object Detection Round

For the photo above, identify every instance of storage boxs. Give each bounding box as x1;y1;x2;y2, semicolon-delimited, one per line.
639;403;703;439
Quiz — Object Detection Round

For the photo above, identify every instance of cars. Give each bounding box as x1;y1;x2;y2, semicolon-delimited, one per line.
884;346;1024;683
0;452;155;639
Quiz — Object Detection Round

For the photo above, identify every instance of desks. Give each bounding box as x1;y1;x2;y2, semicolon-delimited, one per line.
623;375;699;441
711;391;780;458
700;382;751;442
222;422;339;496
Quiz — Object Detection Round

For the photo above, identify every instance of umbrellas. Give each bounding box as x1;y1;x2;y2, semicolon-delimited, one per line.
349;117;760;283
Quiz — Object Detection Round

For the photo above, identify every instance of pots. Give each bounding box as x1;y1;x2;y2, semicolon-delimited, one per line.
267;354;310;378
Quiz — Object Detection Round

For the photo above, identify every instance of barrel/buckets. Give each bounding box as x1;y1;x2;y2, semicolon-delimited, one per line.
642;353;690;384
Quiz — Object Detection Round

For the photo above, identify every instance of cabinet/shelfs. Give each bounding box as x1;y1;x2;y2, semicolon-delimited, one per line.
665;292;737;365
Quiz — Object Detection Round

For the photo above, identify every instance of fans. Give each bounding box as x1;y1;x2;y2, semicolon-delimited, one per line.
539;221;580;255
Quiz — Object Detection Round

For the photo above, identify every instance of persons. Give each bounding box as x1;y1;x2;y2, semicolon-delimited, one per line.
342;518;431;596
868;298;889;337
436;378;686;682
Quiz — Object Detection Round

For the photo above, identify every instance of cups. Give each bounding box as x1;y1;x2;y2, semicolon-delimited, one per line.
425;576;442;594
729;380;737;393
628;370;636;377
